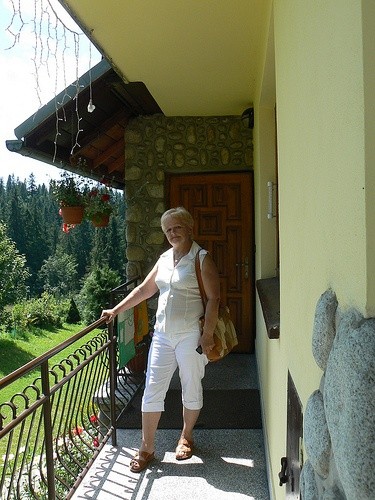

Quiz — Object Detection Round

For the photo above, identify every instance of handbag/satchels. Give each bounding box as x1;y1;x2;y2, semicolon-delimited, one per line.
198;303;238;362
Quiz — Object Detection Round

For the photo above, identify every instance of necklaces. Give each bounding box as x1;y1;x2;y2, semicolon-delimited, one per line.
174;250;188;263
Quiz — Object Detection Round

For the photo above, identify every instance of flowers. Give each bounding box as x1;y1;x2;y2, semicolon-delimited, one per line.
48;170;124;237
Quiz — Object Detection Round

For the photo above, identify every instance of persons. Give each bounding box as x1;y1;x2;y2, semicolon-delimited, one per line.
100;206;221;473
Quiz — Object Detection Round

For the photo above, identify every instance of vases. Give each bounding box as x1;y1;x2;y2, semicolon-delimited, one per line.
91;213;110;227
61;205;84;225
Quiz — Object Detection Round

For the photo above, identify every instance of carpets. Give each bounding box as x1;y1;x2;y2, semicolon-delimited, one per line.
117;388;263;430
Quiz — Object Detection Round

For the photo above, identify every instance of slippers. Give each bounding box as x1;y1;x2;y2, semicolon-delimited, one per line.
129;451;155;473
174;439;194;459
